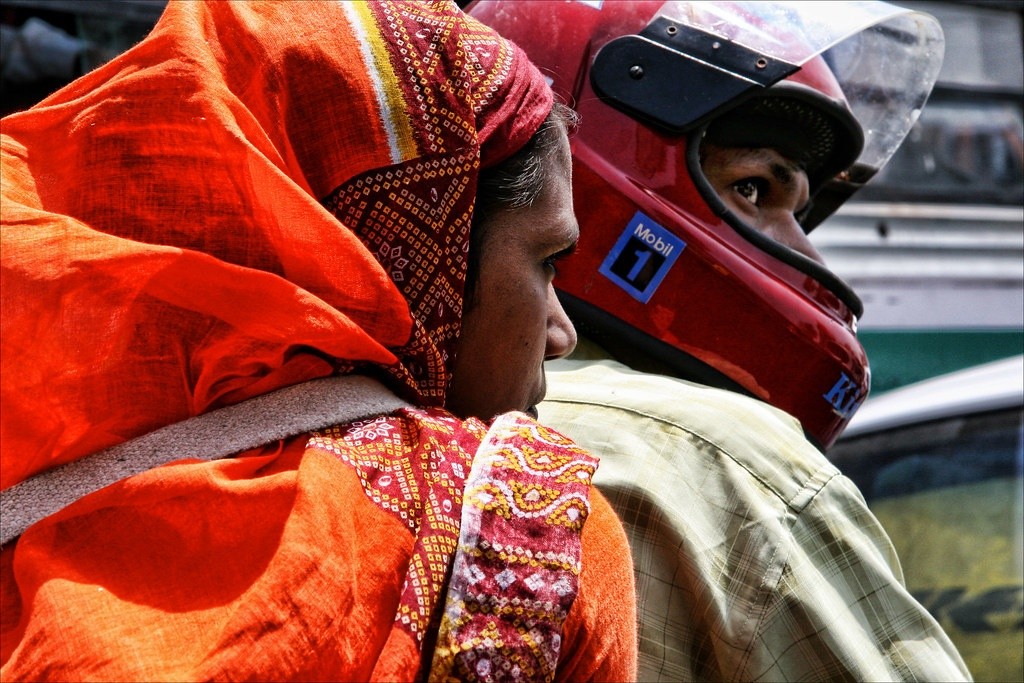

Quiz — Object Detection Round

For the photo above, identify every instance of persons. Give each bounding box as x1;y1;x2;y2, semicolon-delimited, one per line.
455;0;975;683
0;0;640;683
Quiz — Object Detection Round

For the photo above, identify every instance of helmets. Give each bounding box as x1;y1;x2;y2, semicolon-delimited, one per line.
459;0;947;454
